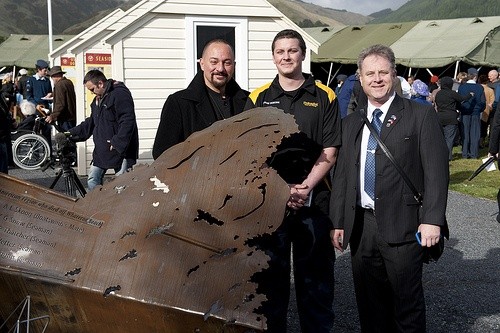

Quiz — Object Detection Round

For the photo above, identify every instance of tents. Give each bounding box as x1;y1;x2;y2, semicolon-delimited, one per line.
303;16;500;86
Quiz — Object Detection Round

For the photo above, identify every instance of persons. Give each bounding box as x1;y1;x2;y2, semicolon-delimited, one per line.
485;69;500;147
394;75;441;112
45;66;77;166
335;69;358;119
329;44;449;333
68;69;140;190
458;68;486;160
0;68;28;174
487;96;500;223
436;76;474;160
241;29;342;333
478;75;495;149
152;39;250;227
26;60;56;165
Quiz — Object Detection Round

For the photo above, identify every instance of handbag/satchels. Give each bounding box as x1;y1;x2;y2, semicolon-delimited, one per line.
414;191;450;263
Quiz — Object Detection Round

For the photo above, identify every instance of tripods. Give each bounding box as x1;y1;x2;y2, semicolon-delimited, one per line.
48;167;87;199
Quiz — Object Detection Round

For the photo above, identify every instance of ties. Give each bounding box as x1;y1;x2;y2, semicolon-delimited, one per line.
363;109;382;200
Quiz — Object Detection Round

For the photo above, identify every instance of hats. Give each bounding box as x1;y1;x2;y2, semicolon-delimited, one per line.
35;59;48;68
48;65;66;77
468;67;479;75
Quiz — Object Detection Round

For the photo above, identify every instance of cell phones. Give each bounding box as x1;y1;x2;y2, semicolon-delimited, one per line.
415;232;422;245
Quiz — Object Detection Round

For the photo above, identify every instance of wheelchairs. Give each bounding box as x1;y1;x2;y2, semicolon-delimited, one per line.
12;115;51;170
